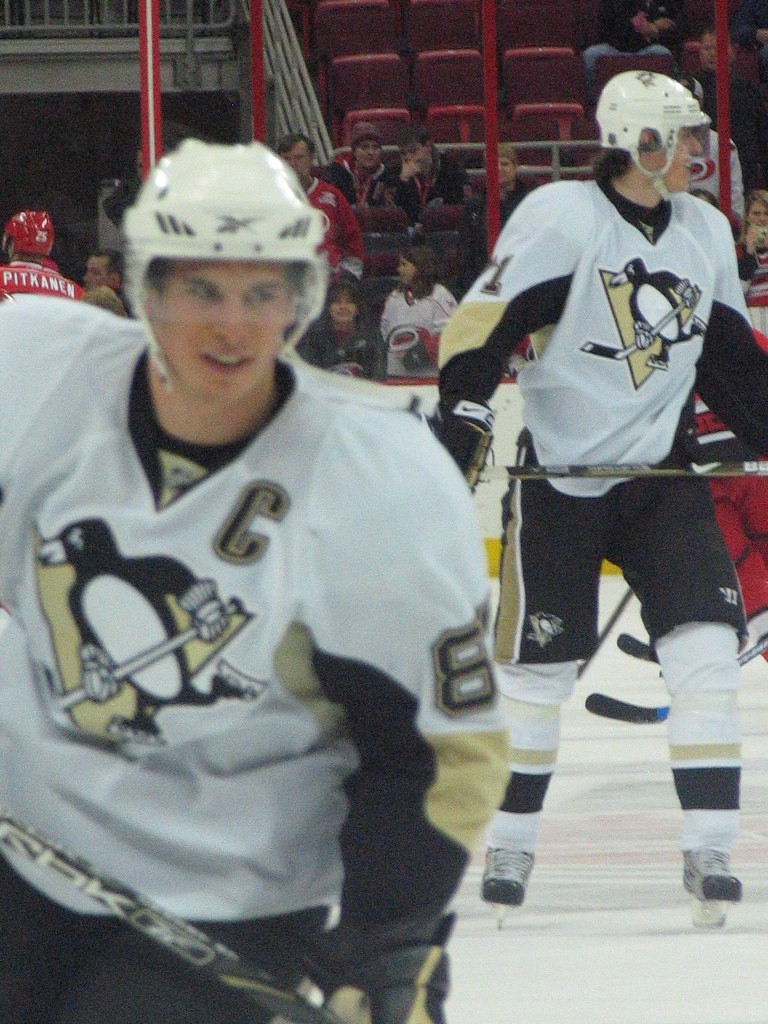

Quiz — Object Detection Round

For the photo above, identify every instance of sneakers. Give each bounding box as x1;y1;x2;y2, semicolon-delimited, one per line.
479;844;534;928
683;847;742;929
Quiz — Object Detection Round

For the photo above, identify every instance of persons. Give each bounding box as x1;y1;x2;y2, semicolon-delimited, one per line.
0;212;82;298
82;140;174;317
436;70;768;930
696;325;767;662
578;0;768;307
0;138;513;1023
278;134;523;381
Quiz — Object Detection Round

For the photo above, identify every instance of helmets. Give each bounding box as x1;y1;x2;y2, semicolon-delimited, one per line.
2;210;55;254
119;137;330;325
596;73;713;151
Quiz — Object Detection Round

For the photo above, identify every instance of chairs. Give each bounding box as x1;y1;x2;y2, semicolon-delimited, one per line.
289;0;768;319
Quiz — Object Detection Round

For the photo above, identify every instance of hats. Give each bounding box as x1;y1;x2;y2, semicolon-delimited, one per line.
349;123;382;148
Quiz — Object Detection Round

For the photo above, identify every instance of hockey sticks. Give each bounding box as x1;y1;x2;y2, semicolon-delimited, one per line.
505;463;767;477
585;635;768;725
2;813;335;1024
613;635;660;663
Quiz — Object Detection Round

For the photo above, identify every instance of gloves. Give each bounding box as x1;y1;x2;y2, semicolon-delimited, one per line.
428;394;492;493
